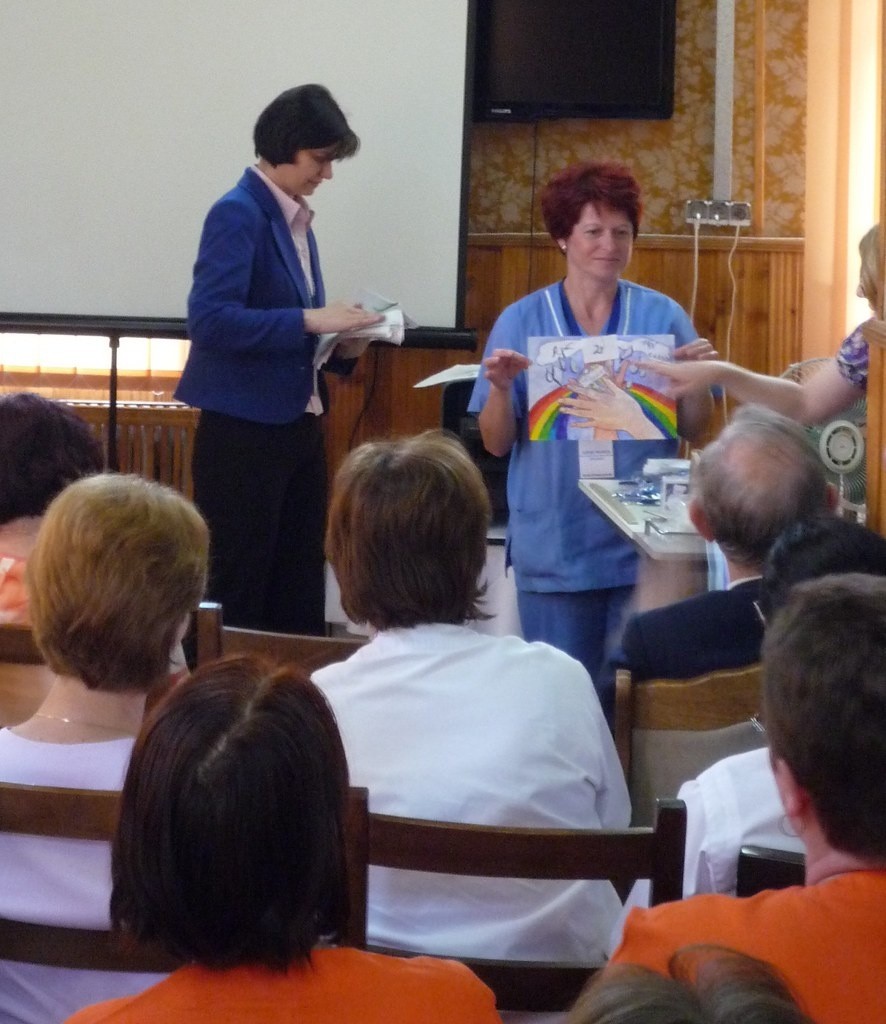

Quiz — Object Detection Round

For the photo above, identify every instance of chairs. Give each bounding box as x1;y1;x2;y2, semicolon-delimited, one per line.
0;601;807;1011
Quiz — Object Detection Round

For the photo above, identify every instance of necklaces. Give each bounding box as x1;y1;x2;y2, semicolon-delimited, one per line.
35;713;135;737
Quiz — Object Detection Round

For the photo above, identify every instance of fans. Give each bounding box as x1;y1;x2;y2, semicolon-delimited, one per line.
780;356;867;522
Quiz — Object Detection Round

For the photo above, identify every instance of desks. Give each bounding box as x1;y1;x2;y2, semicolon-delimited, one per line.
578;479;711;610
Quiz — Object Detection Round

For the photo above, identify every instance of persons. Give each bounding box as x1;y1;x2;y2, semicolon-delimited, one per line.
0;390;886;1024
465;161;724;689
646;219;884;427
171;83;385;639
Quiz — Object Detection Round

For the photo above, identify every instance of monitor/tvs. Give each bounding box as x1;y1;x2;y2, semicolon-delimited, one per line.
472;0;676;120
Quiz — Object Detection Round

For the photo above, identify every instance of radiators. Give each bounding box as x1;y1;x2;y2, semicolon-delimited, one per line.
52;398;194;487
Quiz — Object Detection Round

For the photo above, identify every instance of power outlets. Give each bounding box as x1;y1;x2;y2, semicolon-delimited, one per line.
686;200;752;227
